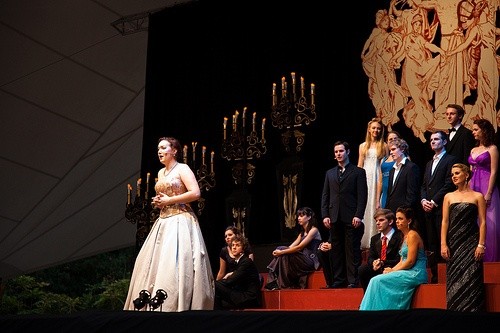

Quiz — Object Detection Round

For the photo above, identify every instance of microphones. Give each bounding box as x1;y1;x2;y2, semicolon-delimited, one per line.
165;152;174;157
334;157;337;159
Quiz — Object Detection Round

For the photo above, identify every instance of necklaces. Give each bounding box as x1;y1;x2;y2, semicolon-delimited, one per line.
164;162;178;176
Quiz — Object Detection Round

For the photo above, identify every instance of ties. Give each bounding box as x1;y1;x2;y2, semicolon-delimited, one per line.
380;235;387;263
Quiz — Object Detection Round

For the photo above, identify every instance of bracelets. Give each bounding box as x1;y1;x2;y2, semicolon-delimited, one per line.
478;244;486;249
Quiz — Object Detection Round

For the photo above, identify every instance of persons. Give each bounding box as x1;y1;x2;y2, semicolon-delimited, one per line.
469;119;500;262
362;209;402;293
320;222;364;287
384;139;421;227
322;141;369;287
217;226;254;280
376;132;410;209
357;118;386;249
439;163;487;312
420;131;456;283
265;207;323;291
359;206;429;310
444;104;476;171
215;237;263;309
123;137;217;312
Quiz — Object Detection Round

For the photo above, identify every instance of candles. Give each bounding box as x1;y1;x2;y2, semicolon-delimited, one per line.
223;106;266;142
271;73;315;106
127;172;159;206
182;142;215;173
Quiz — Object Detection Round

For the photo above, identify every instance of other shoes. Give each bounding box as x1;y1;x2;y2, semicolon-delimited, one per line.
264;279;278;290
320;285;329;289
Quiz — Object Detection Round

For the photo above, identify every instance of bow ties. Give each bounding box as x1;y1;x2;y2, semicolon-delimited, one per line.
449;128;456;133
